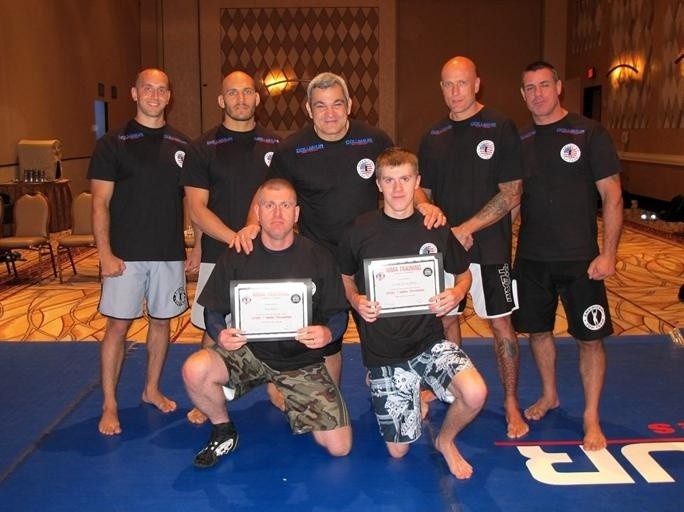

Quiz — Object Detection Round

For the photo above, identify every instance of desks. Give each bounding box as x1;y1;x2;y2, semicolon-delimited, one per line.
4;180;73;233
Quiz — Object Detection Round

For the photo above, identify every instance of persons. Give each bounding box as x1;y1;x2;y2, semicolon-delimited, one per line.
224;70;449;397
411;49;532;441
179;174;354;471
333;142;491;480
178;68;286;428
509;58;628;456
83;65;203;440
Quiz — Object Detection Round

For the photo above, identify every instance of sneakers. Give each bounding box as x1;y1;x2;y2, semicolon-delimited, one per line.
194;430;239;467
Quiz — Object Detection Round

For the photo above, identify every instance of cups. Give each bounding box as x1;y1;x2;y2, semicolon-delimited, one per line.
22;167;45;182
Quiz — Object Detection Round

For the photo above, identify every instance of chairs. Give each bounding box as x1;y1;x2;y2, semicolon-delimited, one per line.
0;191;56;289
55;183;105;281
